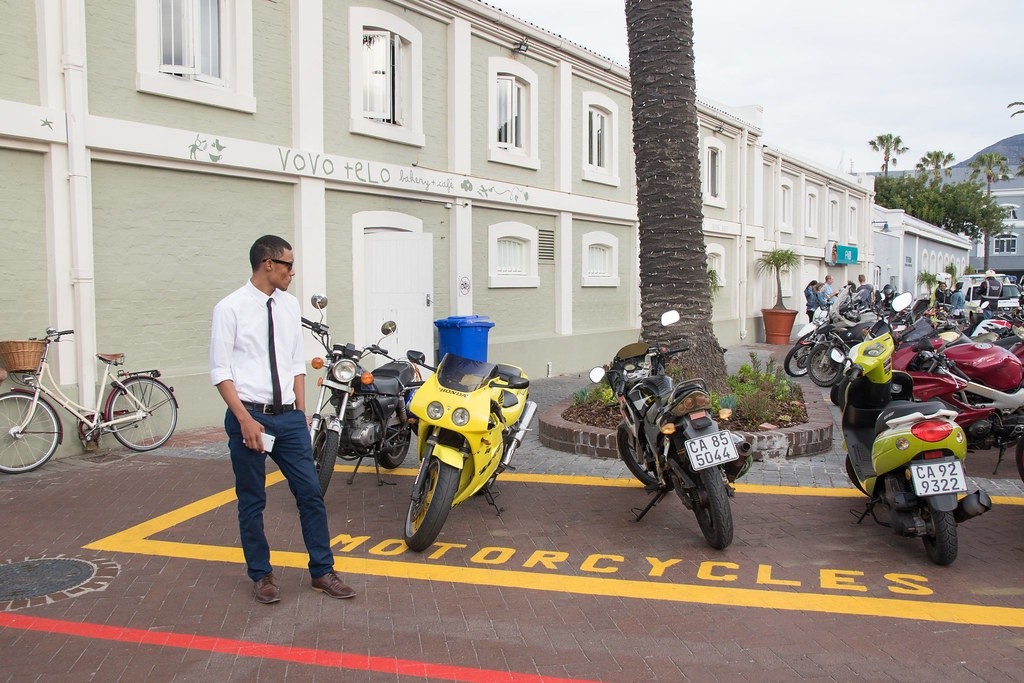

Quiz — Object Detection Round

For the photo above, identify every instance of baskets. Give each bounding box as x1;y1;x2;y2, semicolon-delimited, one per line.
0;340;45;372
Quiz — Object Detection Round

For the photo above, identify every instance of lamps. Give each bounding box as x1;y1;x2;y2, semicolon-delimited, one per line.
512;41;528;51
872;221;892;233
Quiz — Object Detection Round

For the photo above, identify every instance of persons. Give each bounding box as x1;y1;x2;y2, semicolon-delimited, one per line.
929;272;966;325
209;235;358;604
975;270;1002;320
804;274;900;322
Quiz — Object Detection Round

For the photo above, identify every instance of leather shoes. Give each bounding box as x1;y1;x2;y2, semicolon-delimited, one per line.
311;571;356;598
251;571;280;603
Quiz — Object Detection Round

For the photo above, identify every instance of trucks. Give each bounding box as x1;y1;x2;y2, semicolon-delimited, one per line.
958;274;1018;284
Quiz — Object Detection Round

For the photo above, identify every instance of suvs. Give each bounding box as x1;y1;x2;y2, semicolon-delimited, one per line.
965;285;1021;324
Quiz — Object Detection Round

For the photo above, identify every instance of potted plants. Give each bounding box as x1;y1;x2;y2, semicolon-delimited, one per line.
755;247;800;346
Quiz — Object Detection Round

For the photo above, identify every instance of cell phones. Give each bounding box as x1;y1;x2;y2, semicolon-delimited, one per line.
243;432;276;453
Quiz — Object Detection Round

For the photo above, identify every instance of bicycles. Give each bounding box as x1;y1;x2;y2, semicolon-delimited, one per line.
1;328;179;475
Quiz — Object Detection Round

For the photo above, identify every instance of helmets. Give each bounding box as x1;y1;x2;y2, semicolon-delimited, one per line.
985;270;995;279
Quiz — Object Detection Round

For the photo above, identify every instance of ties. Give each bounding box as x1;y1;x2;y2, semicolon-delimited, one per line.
266;298;282;414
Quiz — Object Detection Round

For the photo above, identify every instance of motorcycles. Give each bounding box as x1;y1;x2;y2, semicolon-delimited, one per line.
590;309;755;546
301;293;423;496
831;292;993;566
893;317;1024;485
401;349;537;554
782;283;1024;383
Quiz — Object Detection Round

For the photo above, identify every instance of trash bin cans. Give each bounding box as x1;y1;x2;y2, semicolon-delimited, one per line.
434;314;496;364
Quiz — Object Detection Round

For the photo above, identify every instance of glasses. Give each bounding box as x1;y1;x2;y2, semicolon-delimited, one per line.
262;258;292;271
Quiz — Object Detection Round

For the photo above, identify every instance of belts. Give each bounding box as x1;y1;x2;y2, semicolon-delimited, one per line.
240;401;294;415
